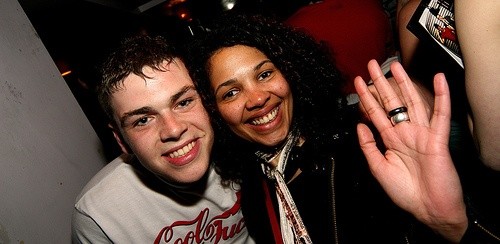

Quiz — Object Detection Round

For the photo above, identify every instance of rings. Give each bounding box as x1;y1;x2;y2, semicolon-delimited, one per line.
387;104;410;128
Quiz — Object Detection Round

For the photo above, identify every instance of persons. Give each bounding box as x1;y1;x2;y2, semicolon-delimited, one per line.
392;2;498;171
199;26;477;241
71;38;256;244
